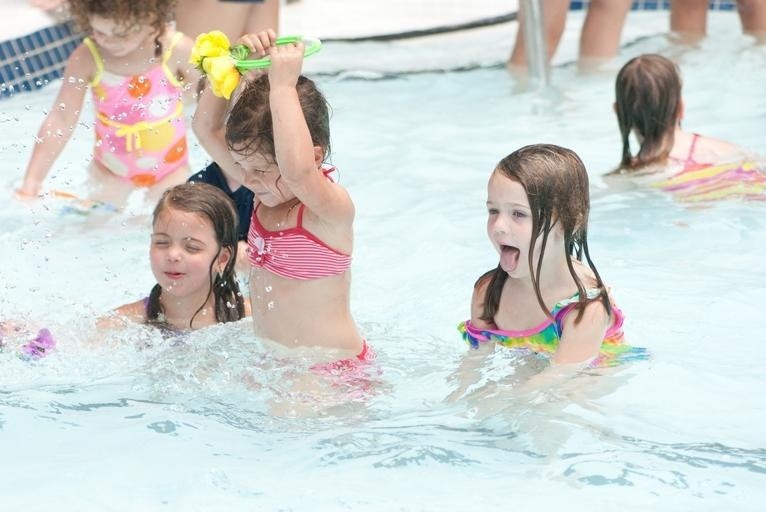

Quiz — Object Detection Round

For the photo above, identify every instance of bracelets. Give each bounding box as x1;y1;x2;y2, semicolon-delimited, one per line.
15;188;37;199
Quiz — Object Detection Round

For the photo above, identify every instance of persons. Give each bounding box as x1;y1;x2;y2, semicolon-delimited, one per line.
12;0;208;229
442;141;633;423
0;179;255;370
188;27;386;421
599;52;766;209
505;1;635;97
173;0;282;99
185;159;257;247
667;2;765;50
